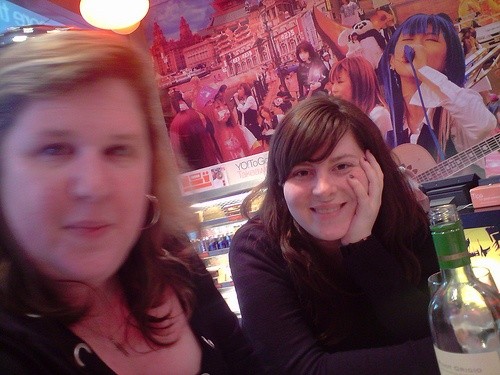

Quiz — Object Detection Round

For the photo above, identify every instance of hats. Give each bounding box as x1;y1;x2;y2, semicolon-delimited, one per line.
192;81;227;113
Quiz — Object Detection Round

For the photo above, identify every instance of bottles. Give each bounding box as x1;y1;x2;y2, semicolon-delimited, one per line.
427;204;500;374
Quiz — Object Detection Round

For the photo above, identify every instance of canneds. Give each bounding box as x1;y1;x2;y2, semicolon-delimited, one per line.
189;231;235;252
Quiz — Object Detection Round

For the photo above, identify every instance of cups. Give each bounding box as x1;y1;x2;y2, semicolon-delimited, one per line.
427;266;500;302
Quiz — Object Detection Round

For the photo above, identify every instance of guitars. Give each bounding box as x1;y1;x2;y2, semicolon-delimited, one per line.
392;132;500;185
272;96;296;107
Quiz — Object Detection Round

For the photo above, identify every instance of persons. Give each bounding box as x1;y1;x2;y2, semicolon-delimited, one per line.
228;96;466;375
331;0;500;216
170;39;336;174
0;28;256;374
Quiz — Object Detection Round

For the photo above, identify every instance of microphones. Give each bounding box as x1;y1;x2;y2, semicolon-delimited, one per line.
404;44;415;64
229;96;234;102
